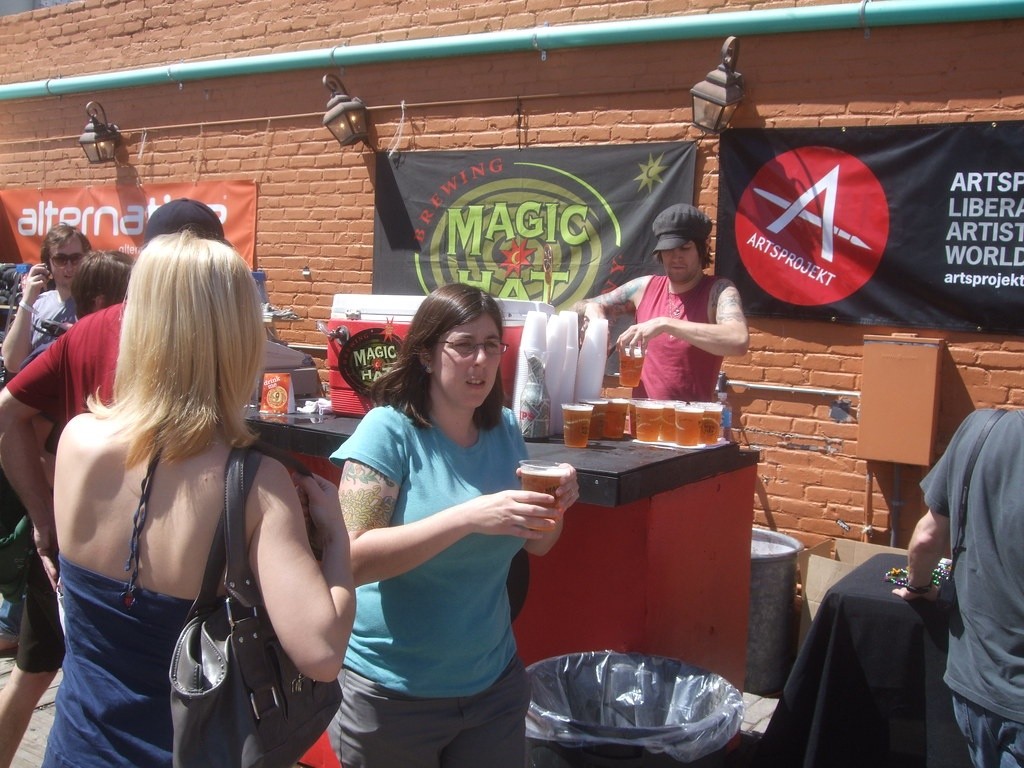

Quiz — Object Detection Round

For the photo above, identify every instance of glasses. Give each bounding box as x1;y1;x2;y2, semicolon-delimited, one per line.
48;253;83;267
430;339;510;354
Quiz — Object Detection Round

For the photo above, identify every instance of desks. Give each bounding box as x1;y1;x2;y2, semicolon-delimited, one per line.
238;398;756;768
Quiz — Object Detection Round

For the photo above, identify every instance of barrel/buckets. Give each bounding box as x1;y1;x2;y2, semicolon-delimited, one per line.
744;527;805;696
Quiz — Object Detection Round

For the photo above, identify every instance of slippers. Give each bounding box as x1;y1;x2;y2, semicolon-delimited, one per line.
0;646;20;658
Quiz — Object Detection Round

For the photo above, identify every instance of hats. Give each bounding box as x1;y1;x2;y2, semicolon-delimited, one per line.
650;203;712;254
144;198;224;243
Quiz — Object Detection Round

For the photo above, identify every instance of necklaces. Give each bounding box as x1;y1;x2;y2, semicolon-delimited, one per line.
884;562;950;587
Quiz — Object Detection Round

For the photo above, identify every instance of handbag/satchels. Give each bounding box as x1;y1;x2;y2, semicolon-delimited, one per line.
172;442;343;768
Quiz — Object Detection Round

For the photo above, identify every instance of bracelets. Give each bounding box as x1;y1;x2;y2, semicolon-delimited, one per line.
908;586;932;593
19;300;34;313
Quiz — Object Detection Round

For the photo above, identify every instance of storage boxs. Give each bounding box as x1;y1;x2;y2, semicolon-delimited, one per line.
797;536;909;662
327;293;557;420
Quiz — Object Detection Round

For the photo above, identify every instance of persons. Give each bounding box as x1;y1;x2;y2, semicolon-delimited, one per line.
41;230;356;768
567;203;750;434
0;198;233;592
0;224;136;768
332;283;579;768
891;408;1024;768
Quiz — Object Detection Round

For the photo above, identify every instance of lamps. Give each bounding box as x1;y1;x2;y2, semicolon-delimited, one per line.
688;36;748;136
75;99;124;166
320;71;373;149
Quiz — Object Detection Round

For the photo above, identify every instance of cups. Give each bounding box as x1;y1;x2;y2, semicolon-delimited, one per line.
619;345;645;387
608;398;722;446
519;460;562;531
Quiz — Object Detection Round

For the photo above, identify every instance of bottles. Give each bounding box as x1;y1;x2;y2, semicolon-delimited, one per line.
520;348;551;439
512;311;608;448
715;393;731;444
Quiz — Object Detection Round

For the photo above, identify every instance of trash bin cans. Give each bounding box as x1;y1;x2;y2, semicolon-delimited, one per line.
526;651;735;768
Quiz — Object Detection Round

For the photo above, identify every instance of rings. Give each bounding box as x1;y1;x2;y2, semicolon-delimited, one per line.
571;489;574;497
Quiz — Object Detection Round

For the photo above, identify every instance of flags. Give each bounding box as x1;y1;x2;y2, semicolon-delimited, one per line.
371;141;697;377
714;120;1024;336
0;179;256;273
